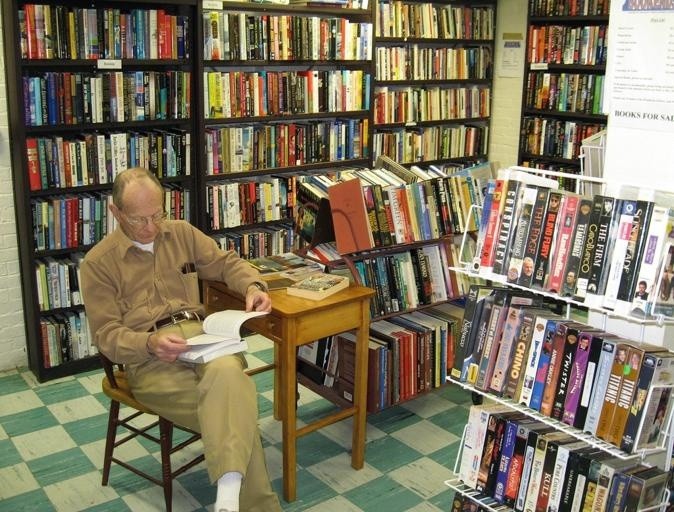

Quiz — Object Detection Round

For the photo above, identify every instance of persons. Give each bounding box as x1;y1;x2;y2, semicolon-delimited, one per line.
76;163;286;511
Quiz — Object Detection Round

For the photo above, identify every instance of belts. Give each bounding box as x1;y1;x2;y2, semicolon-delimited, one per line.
146;311;204;332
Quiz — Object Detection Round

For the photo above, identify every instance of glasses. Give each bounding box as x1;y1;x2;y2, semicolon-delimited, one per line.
121;211;169;225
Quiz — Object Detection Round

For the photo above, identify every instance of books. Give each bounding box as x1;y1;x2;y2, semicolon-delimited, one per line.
471;174;674;318
17;5;193;369
451;405;668;512
520;0;611;192
374;1;495;174
179;310;268;363
203;0;372;260
246;166;507;411
450;284;673;454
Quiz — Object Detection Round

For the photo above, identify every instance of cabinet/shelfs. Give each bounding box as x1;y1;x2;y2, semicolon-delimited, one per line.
517;1;610;195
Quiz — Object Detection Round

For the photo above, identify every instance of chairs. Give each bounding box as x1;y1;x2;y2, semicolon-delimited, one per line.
98;353;206;512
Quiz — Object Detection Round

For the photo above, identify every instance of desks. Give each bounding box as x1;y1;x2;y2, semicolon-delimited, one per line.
200;272;378;504
